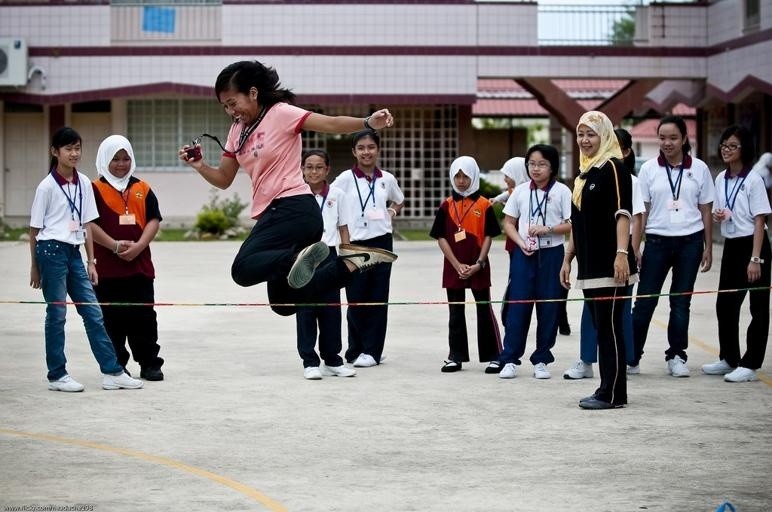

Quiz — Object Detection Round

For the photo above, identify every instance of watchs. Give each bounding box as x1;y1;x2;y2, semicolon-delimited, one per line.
546;225;552;235
750;256;764;264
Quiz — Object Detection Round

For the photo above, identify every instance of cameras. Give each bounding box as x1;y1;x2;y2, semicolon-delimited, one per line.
184;146;203;163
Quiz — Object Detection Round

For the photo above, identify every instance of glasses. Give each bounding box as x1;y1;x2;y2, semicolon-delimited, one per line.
719;144;741;150
527;162;547;168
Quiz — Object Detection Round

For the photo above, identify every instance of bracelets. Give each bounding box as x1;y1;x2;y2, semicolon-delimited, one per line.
388;208;397;218
114;239;120;253
86;258;97;265
475;260;486;271
364;116;370;131
616;249;629;255
566;251;576;257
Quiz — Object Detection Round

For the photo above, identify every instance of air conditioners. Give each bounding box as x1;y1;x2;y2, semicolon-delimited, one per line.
0;36;29;90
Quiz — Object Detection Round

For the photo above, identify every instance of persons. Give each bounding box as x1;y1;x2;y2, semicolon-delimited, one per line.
702;125;771;382
429;156;505;374
29;126;143;393
626;117;716;376
751;152;772;245
89;134;165;381
329;129;407;367
489;156;569;336
559;110;633;409
564;129;646;379
298;149;358;380
178;61;396;315
498;144;572;377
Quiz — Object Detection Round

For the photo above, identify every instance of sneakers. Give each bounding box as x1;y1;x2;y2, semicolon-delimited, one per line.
669;355;688;377
304;353;377;379
441;361;516;378
626;365;640;374
533;362;550;379
48;375;84;392
704;359;758;381
579;394;624;409
339;243;397;274
103;369;163;389
563;361;593;379
288;242;330;288
